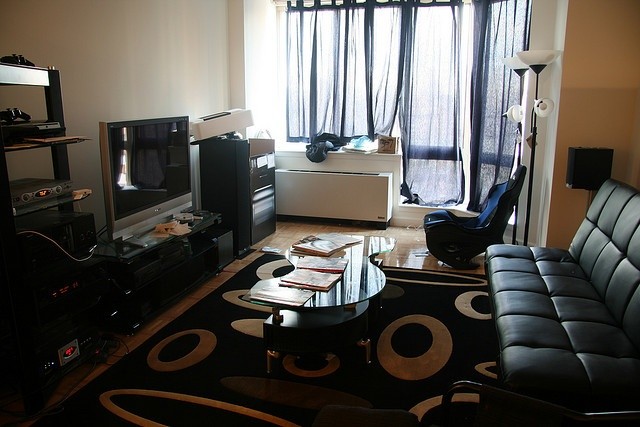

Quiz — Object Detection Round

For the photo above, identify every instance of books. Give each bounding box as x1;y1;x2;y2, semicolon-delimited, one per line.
291;232;362;257
297;257;350;273
277;268;340;292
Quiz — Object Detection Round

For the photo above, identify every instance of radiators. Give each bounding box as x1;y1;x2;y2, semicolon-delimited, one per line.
275;169;393;230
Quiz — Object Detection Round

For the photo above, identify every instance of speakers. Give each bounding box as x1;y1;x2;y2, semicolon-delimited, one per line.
199;141;250;256
564;146;614;192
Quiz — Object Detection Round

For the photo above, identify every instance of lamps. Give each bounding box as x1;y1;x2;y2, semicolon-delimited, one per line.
516;50;563;245
502;57;530;245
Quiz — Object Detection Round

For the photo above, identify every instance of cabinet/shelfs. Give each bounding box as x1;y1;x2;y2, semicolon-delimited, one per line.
89;206;236;336
200;138;276;256
1;62;95;261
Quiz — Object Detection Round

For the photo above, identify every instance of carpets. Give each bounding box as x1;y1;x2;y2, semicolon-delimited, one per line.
32;254;502;426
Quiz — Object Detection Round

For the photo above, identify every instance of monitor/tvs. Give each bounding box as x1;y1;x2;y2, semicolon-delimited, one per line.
98;115;193;248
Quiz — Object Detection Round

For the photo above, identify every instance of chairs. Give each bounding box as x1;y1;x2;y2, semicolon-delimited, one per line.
423;166;527;269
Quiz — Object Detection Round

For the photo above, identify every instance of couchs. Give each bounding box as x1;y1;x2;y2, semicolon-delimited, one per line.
316;379;640;427
484;179;640;412
